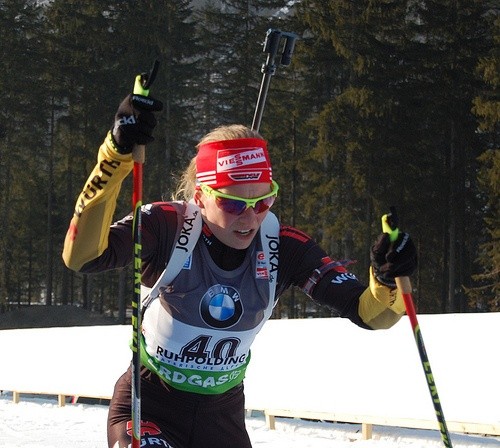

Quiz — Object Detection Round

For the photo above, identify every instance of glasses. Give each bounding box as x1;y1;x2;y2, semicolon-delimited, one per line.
198;181;280;216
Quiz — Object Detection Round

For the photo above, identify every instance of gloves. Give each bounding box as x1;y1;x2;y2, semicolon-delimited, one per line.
111;95;164;154
369;231;417;287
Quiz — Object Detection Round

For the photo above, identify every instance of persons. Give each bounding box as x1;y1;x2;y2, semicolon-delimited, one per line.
62;93;418;448
